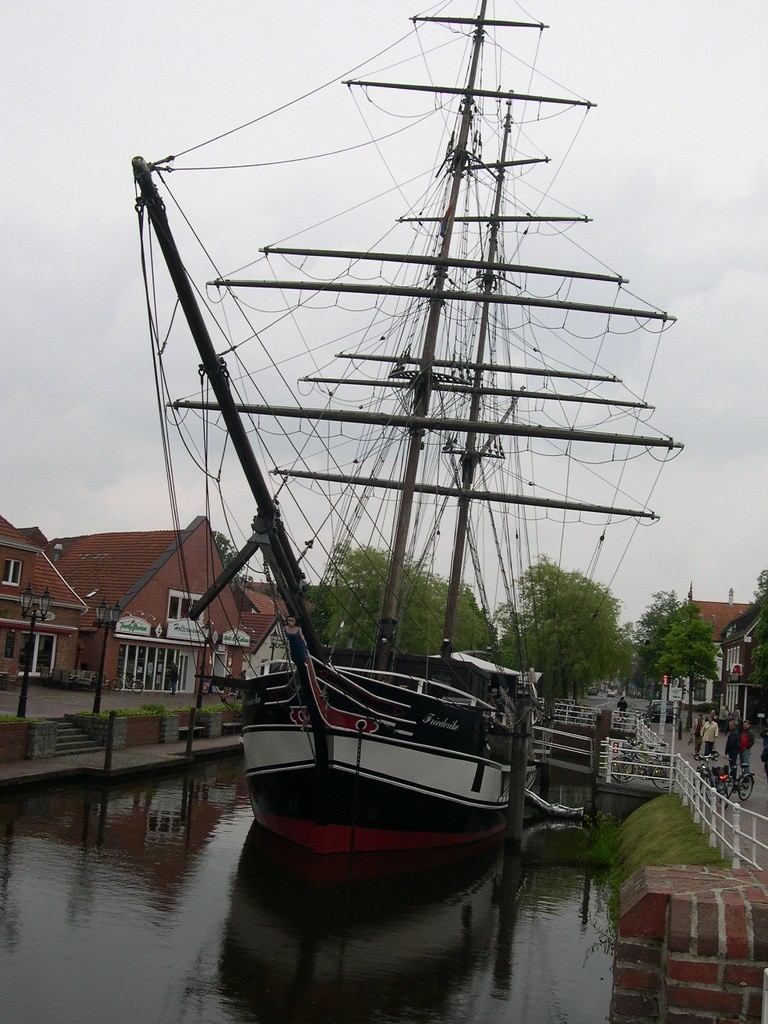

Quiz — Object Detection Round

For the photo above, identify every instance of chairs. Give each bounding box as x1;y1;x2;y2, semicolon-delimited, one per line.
0;666;108;692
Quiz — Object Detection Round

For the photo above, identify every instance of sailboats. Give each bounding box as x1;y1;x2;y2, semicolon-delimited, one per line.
123;0;689;858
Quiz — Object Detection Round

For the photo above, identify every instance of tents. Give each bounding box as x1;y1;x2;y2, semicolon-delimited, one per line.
430;652;518;713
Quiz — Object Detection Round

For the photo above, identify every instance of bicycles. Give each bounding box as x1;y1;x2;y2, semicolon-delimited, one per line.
611;736;678;790
692;749;755;810
613;705;653;734
554;697;595;724
111;666;144;694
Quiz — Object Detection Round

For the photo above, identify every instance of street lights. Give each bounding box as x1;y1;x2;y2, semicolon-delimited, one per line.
195;624;220;708
91;594;125;712
13;582;56;718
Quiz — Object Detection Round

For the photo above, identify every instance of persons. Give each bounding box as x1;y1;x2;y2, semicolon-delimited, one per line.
169;663;179;695
617;697;627;716
221;669;246;698
689;700;768;781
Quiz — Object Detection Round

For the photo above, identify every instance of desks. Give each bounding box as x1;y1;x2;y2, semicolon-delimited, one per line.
0;672;15;689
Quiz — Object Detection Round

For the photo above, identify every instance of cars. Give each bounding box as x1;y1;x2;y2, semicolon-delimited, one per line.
647;703;674;722
607;691;616;698
646;699;668;708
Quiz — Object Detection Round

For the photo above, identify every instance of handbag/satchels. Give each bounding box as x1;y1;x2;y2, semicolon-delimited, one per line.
694;730;700;736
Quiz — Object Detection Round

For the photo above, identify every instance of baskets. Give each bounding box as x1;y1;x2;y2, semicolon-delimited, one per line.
622;742;633;754
